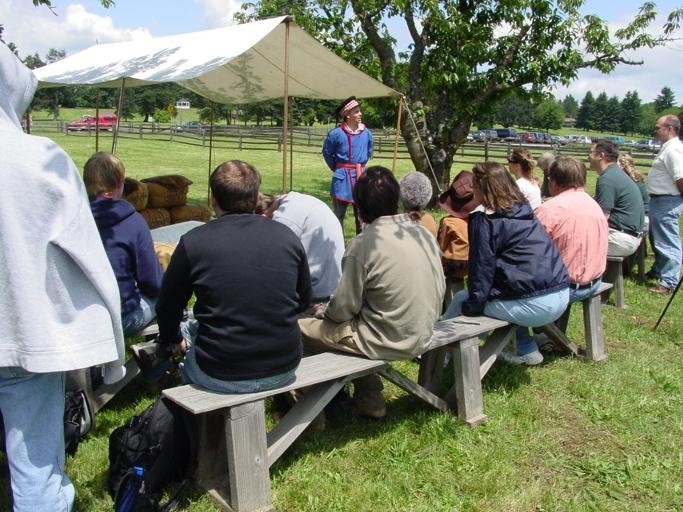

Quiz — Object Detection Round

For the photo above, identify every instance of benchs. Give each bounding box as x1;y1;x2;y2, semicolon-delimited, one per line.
605;216;648;310
60;306;195;430
161;278;614;511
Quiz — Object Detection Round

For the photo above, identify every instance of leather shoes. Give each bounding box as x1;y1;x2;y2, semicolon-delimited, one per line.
568;277;600;289
647;284;669;293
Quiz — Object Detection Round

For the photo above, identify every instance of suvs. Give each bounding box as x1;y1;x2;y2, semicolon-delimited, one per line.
67;115;117;131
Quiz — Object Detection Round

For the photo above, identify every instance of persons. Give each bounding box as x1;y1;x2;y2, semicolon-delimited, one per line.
529;153;609;356
586;137;645;304
617;154;650;238
253;191;346;302
412;161;570;372
399;170;437;244
432;170;479;314
538;151;558;200
0;41;127;511
144;160;311;398
643;114;682;294
82;149;163;335
508;146;541;213
323;95;373;238
294;166;446;433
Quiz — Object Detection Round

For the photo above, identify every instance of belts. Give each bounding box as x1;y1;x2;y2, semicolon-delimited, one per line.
623;229;638;237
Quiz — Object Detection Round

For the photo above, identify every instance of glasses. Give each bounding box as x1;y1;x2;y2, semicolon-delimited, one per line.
507;156;514;164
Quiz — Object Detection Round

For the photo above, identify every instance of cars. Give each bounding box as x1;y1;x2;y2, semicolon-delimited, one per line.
624;139;662;152
172;121;211;133
466;129;623;146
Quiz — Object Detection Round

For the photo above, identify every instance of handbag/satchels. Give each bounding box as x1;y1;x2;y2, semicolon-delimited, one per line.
64;388;94;459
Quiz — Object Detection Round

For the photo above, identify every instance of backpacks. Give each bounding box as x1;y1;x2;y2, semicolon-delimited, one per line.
109;395;200;512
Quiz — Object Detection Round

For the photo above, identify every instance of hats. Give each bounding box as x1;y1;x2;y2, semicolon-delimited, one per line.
336;96;360;118
438;170;482;218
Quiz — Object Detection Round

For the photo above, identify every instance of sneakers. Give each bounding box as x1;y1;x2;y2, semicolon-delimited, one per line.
415;352;450;368
130;338;178;376
533;333;554;352
312;414;326;433
502;350;544;365
353;407;386;421
